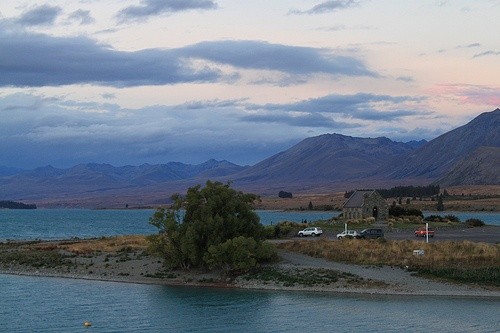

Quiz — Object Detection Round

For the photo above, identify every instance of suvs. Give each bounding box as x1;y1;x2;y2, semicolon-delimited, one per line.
354;228;384;240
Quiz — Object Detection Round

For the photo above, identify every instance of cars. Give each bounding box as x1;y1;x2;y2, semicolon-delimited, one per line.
414;227;435;238
337;230;359;240
297;227;323;238
